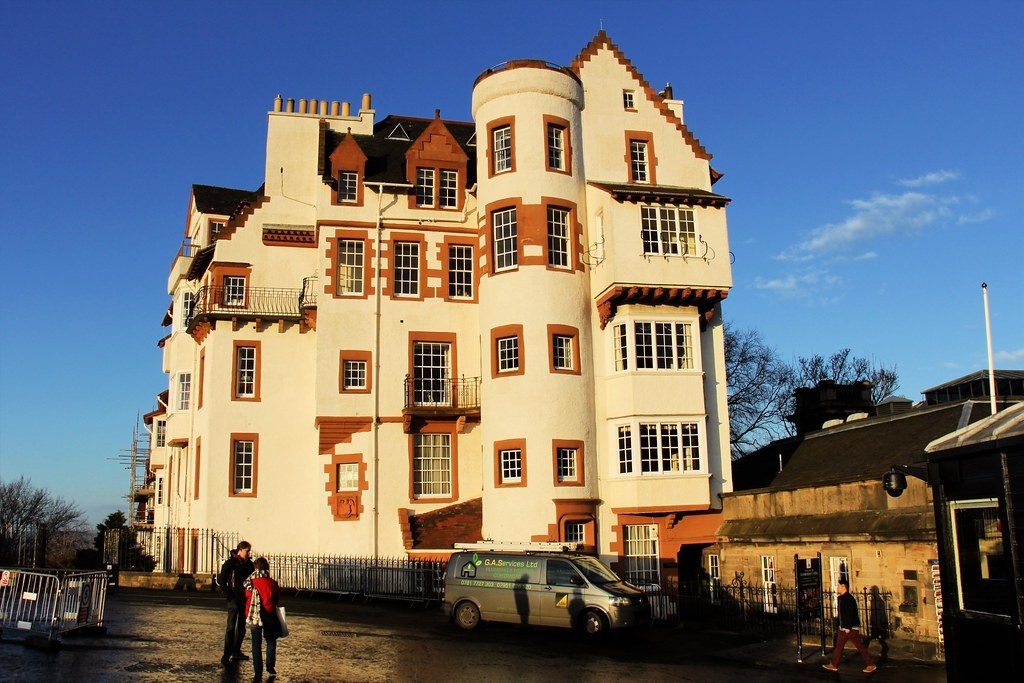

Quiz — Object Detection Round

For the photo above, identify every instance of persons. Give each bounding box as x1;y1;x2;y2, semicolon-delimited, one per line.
244;556;282;682
218;540;255;664
822;578;877;673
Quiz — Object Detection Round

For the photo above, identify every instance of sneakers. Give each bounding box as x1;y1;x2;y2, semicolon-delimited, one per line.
822;663;838;671
863;665;876;672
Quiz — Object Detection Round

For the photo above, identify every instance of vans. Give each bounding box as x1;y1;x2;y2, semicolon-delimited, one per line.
440;551;653;644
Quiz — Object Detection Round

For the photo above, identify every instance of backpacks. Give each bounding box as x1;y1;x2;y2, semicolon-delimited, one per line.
212;574;228;594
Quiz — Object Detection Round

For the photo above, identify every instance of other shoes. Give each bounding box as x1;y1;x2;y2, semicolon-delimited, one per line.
253;672;262;682
221;653;249;664
267;667;276;674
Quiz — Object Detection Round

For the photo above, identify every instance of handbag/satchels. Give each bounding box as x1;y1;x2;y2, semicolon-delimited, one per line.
276;607;289;638
